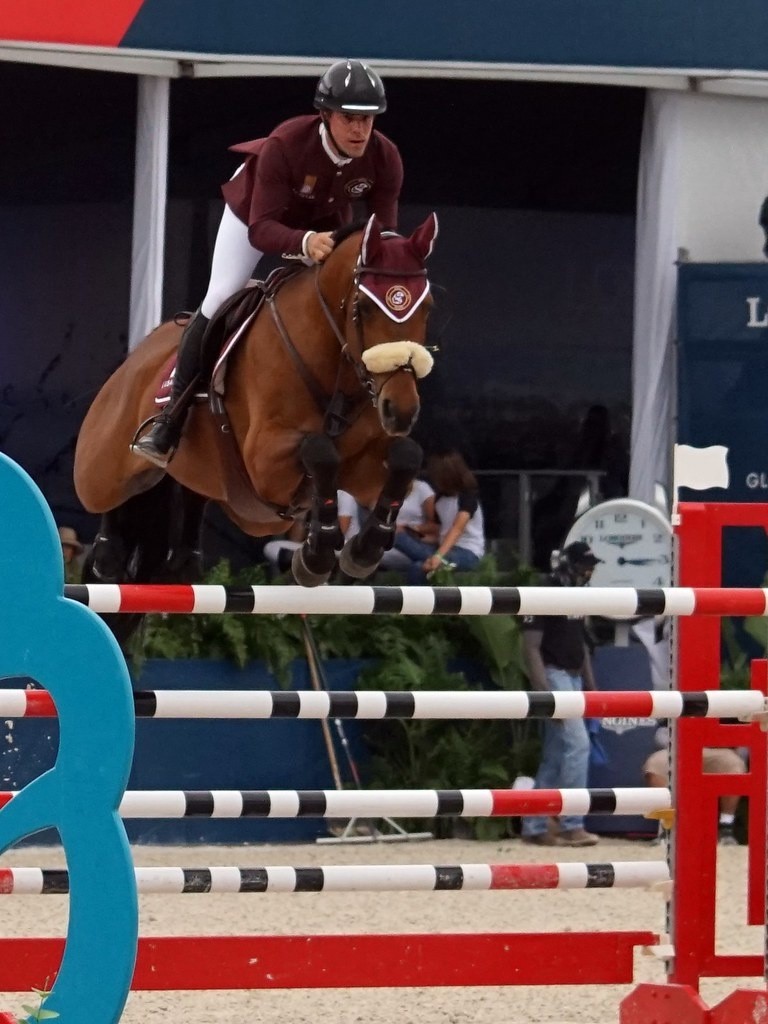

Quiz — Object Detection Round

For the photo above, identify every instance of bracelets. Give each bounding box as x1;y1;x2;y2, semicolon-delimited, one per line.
435;551;443;559
719;813;735;834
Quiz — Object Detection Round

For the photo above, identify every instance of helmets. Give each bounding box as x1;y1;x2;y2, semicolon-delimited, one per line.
314;59;387;114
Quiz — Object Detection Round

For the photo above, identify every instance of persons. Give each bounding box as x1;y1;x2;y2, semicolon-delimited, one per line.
129;61;404;467
644;747;747;846
522;541;602;848
263;454;485;572
58;527;85;584
565;403;630;501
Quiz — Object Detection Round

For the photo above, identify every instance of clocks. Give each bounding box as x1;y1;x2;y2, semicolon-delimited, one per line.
562;498;672;627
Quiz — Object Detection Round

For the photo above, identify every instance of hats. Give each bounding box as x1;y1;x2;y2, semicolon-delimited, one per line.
559;541;600;571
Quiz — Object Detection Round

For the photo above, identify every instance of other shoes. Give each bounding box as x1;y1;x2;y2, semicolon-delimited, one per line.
523;826;599;845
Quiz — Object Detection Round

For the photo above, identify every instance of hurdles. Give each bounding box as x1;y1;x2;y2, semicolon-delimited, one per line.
0;451;768;1024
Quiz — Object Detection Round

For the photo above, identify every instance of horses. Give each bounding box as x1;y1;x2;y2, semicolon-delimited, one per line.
62;207;440;638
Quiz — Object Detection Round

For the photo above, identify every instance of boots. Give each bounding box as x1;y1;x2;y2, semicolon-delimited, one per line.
129;302;223;469
